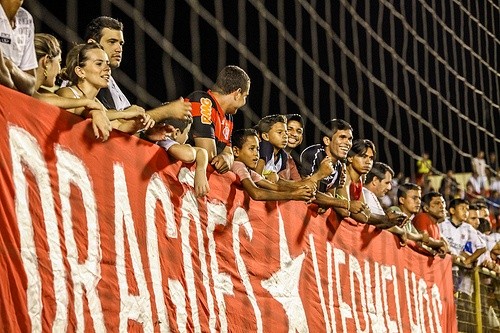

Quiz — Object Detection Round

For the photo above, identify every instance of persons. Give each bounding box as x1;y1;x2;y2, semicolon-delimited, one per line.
334;158;372;223
228;129;317;203
377;152;500;333
299;118;367;218
253;115;289;184
362;162;407;247
136;102;210;197
31;34;112;142
183;65;249;174
277;113;334;185
1;0;39;96
344;139;409;231
53;42;156;136
53;16;192;135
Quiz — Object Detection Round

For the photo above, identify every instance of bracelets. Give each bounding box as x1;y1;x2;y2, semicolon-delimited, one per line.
347;200;351;210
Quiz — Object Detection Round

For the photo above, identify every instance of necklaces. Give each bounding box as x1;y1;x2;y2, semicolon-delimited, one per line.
75;84;86;97
